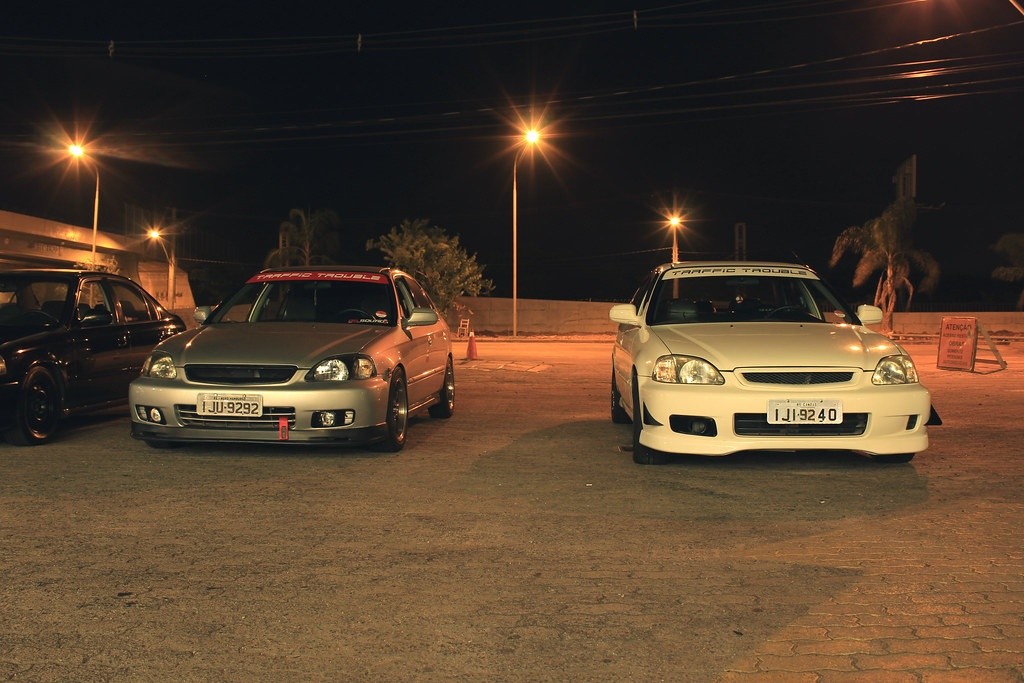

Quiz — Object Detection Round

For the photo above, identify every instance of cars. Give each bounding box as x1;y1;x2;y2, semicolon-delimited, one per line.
0;266;186;447
607;261;943;466
129;265;455;452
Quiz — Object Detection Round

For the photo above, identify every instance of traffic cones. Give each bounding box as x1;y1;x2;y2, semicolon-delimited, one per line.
465;332;479;360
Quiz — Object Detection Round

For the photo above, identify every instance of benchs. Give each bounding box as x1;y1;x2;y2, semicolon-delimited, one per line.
78;301;135;321
696;296;762;321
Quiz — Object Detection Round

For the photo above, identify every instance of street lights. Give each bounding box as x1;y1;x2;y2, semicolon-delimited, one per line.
513;125;538;336
69;144;99;265
669;212;685;300
149;228;176;314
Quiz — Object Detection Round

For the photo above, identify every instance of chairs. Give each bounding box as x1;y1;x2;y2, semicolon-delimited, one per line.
280;299;317;322
457;319;469;337
667;299;700;323
40;300;66;326
0;302;22;326
